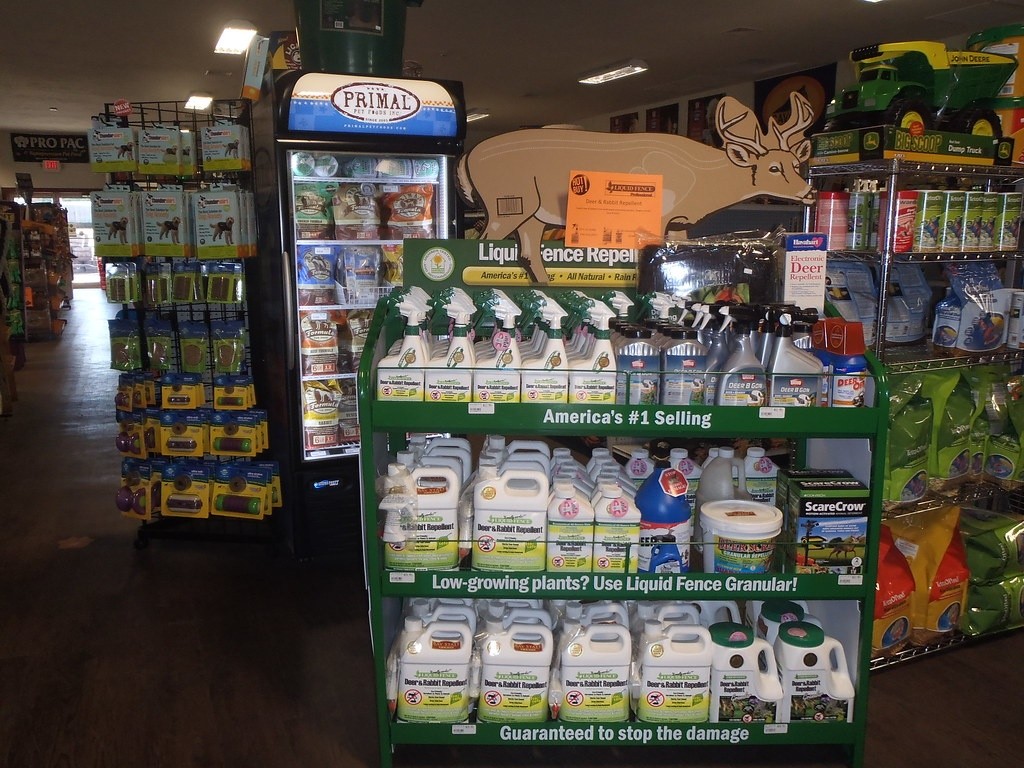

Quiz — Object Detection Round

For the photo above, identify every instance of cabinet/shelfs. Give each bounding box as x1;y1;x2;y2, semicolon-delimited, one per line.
24;202;73;311
0;201;26;371
803;158;1024;670
91;99;283;547
23;221;68;341
356;287;889;768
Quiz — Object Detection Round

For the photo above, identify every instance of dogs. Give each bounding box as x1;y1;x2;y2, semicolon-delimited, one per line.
161;144;178;163
209;217;235;246
104;217;129;245
115;142;134;161
182;145;191;156
156;217;180;244
222;139;240;159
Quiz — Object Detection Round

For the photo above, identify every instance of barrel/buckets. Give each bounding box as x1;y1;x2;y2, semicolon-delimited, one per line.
697;500;784;574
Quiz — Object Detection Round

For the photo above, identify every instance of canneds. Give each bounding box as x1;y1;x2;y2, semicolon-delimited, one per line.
813;190;1024;255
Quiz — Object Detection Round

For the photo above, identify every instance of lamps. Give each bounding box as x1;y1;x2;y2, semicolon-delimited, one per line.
213;19;257;54
467;109;491;123
577;57;649;85
184;92;213;110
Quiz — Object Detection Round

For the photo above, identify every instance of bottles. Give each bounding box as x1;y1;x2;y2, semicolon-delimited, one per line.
392;601;856;723
380;433;787;577
